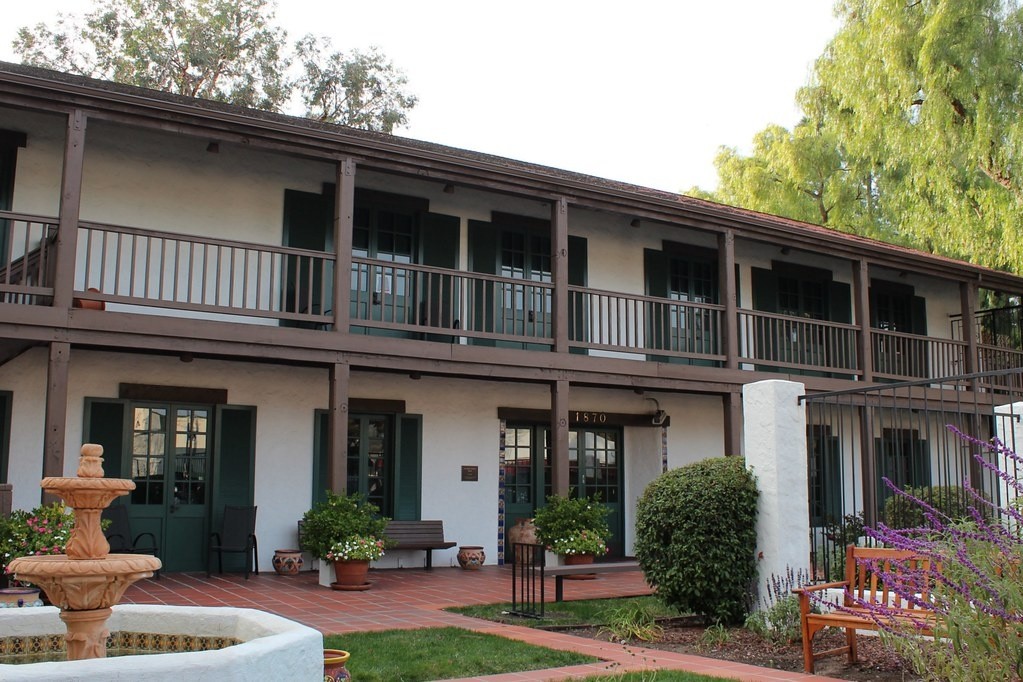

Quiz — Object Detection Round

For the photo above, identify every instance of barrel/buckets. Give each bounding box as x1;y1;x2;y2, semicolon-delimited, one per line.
73;287;106;310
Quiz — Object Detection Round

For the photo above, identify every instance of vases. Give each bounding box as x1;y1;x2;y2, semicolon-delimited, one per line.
564;556;598;579
507;516;543;564
455;546;486;570
272;549;304;576
0;587;45;611
330;559;374;590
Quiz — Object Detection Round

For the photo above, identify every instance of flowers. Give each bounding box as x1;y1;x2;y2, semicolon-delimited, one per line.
1;502;114;566
532;485;614;557
301;491;397;561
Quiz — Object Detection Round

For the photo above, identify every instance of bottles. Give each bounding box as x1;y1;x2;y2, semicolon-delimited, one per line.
508;516;540;565
272;549;304;576
323;648;352;682
456;546;486;570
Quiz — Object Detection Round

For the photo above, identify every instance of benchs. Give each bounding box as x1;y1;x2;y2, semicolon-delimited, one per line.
384;519;458;570
792;542;980;678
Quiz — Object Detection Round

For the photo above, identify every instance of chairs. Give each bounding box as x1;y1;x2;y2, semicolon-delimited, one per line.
101;503;162;580
205;504;260;580
